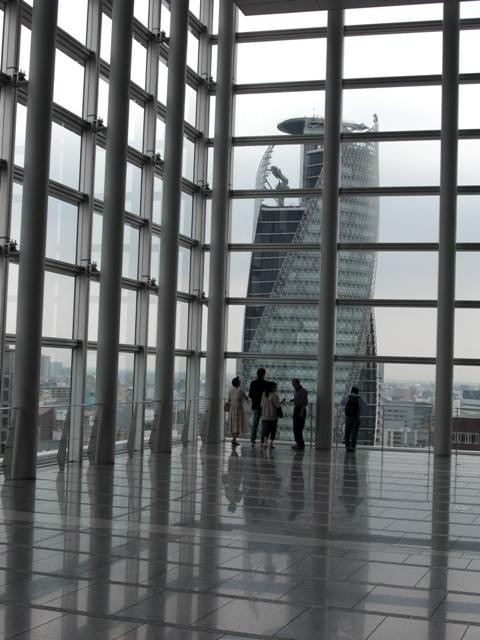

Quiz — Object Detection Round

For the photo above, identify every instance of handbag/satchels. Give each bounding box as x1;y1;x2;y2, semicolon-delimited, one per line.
277;405;284;418
223;401;232;412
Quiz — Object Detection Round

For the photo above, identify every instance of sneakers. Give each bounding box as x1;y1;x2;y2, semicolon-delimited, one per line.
345;447;356;452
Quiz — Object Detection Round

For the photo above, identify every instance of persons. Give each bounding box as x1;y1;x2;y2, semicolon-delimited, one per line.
249;368;270;448
260;381;286;449
340;386;367;452
224;376;249;445
290;378;308;450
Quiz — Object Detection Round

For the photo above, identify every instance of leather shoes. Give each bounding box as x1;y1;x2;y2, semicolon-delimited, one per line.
231;440;305;450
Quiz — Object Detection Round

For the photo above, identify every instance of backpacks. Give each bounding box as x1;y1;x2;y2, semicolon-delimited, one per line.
345;396;360;418
